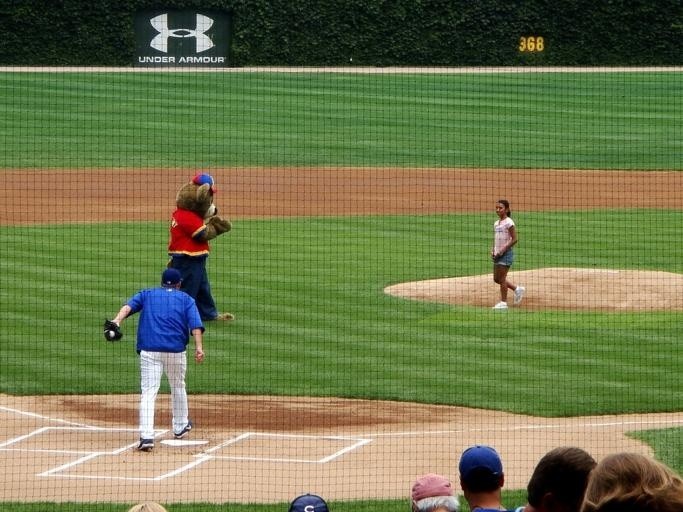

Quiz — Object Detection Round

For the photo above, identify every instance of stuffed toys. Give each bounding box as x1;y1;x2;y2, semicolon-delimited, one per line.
166;173;234;322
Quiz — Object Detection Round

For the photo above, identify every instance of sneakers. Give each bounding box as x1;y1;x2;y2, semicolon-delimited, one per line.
138;438;154;450
175;421;193;439
514;287;524;303
493;302;508;308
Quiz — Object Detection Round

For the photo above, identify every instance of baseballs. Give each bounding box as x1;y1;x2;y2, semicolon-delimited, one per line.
109;331;115;337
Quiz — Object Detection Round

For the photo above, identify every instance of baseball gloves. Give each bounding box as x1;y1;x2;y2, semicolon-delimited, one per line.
104;320;122;340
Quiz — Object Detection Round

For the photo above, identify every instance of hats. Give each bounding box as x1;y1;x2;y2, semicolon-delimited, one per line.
288;494;329;512
412;474;455;502
459;445;502;482
162;270;183;285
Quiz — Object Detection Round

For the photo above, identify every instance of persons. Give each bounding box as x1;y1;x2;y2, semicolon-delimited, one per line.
489;200;526;310
457;445;521;511
580;451;683;511
410;472;460;511
520;445;598;512
103;268;205;451
127;501;167;512
288;492;329;511
